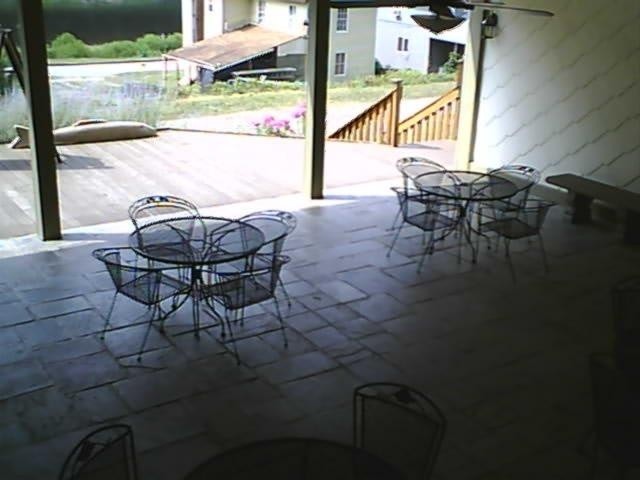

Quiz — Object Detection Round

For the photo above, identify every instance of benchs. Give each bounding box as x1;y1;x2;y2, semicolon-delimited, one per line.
545;174;640;238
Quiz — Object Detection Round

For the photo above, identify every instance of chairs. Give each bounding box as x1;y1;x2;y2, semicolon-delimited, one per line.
353;382;447;480
56;424;138;480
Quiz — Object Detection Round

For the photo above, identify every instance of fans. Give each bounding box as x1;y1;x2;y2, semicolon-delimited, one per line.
329;0;554;18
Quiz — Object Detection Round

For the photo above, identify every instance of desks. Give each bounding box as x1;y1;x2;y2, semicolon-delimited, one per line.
182;437;402;480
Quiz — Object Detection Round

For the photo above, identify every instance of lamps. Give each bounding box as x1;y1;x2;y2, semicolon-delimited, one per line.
410;5;466;33
481;14;498;38
